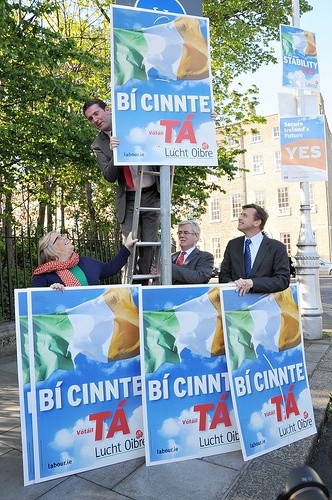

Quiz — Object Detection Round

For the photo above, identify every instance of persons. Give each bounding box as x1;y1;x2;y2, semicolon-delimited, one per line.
30;230;138;292
84;99;161;287
219;204;291;298
172;219;215;286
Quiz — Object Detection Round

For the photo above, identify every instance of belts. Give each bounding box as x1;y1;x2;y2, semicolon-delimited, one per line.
125;182;158;194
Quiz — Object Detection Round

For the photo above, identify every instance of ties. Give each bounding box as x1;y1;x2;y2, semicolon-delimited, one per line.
123;166;136;190
176;251;186;266
244;239;253;275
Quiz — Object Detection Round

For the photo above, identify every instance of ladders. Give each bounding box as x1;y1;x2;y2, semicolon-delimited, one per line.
123;166;175;286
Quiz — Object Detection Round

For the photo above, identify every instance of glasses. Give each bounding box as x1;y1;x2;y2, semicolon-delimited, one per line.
177;231;198;236
52;233;66;245
